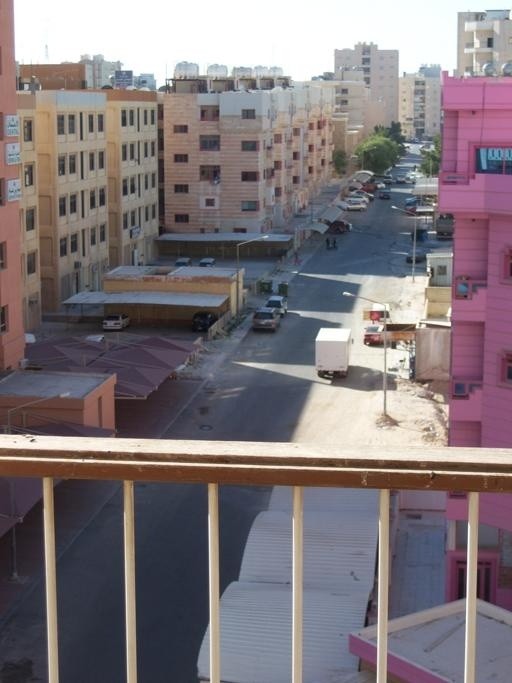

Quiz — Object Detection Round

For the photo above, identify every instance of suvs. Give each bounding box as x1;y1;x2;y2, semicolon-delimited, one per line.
265;294;289;317
199;257;217;266
190;310;216;332
251;305;281;331
174;257;191;267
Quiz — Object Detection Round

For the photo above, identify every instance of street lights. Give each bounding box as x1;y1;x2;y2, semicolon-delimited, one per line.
341;290;388;417
388;204;419;284
7;389;72;579
420;144;433;178
235;233;271;326
361;145;377;167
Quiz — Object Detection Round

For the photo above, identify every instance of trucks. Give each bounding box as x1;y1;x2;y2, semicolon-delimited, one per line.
314;326;356;378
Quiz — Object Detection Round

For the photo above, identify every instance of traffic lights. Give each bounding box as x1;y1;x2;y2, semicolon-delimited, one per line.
370;310;382;322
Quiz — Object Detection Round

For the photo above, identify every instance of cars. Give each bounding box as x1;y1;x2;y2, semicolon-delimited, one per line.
102;312;130;330
362;325;391;345
410;136;420;143
322;167;429;239
405;245;431;263
